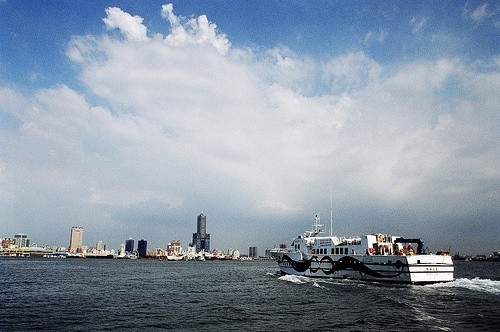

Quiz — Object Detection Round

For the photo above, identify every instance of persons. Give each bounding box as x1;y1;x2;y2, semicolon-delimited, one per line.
419;244;429;253
400;243;414;255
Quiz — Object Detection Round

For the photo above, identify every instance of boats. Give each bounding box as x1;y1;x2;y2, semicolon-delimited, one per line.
269;191;455;285
